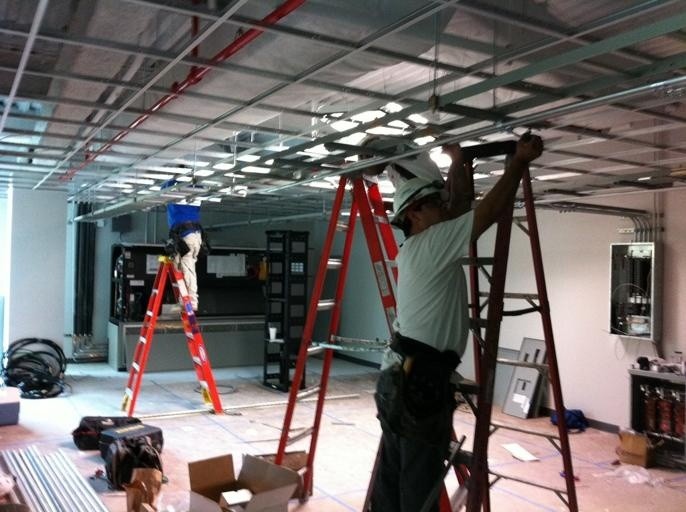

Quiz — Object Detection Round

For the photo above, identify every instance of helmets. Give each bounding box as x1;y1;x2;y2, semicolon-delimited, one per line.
391;177;450;229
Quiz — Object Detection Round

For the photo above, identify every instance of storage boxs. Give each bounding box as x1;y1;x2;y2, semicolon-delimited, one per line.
615;431;665;466
188;454;301;511
1;387;21;427
99;422;164;465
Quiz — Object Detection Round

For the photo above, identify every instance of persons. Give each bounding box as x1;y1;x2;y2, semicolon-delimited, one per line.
322;133;446;194
147;163;203;313
361;134;543;510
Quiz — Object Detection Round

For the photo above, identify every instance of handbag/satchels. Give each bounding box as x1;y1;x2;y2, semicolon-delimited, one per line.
551;407;590;431
104;434;169;492
401;356;449;418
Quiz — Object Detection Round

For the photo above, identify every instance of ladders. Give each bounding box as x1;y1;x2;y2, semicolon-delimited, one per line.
121;256;225;418
428;141;578;512
275;163;470;512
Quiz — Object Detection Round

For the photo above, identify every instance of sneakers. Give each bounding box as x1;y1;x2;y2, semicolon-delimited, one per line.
171;300;200;314
